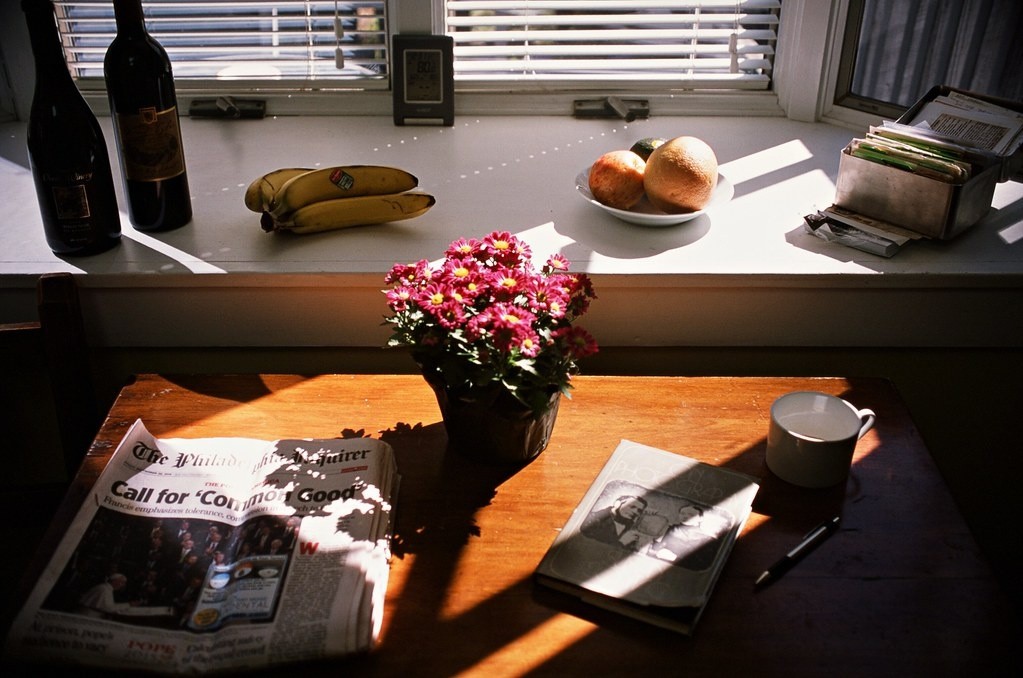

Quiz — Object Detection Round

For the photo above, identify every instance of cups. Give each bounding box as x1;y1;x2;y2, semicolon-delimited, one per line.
766;390;877;489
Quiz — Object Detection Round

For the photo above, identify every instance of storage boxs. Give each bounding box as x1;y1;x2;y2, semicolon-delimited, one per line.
832;84;1023;240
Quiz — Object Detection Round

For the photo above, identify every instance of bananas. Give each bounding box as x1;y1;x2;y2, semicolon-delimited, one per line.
245;165;435;234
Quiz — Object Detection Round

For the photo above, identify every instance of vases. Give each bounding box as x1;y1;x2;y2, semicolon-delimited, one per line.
423;372;561;459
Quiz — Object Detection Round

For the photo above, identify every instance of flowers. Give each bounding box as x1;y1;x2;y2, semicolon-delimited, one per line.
383;231;597;409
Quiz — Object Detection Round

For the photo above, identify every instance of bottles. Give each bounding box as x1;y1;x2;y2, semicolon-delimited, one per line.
103;1;192;232
20;0;123;257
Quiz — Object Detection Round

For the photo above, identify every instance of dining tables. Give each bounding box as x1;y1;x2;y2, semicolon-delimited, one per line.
0;371;1022;678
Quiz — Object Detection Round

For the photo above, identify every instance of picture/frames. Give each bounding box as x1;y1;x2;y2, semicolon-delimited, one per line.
392;35;454;126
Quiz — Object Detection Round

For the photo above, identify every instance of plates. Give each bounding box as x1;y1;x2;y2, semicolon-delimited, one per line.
574;159;735;225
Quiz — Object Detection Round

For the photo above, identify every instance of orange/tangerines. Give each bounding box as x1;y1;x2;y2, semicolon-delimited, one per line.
644;137;718;214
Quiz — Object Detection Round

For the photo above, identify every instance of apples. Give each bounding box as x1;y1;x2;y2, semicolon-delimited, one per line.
590;152;647;210
